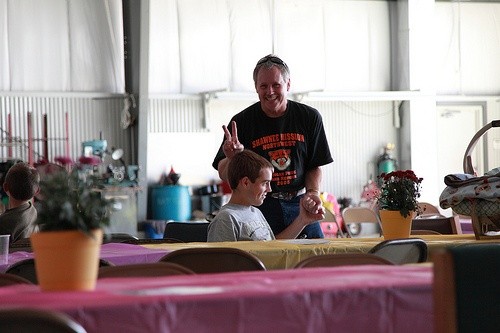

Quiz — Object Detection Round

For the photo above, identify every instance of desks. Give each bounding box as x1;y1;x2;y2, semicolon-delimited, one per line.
0;263;435;333
11;233;500;333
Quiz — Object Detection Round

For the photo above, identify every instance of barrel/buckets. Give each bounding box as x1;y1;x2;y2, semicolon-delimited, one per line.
151;185;191;221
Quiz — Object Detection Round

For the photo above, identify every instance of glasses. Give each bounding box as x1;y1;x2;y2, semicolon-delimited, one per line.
257;56;286;69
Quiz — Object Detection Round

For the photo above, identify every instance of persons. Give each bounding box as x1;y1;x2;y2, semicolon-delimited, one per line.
206;149;326;242
212;54;334;238
0;162;40;244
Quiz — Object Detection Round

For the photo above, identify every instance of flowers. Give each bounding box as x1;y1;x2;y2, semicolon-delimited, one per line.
380;170;419;211
37;157;112;229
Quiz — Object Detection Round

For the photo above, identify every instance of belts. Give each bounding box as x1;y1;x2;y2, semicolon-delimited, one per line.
267;188;306;201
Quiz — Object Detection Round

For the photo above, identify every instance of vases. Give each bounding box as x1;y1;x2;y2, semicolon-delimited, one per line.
381;209;417;239
29;226;104;291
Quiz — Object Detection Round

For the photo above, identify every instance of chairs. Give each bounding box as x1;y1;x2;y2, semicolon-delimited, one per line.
0;200;500;333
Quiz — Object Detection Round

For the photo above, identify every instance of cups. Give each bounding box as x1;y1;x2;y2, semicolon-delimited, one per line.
0;234;10;265
